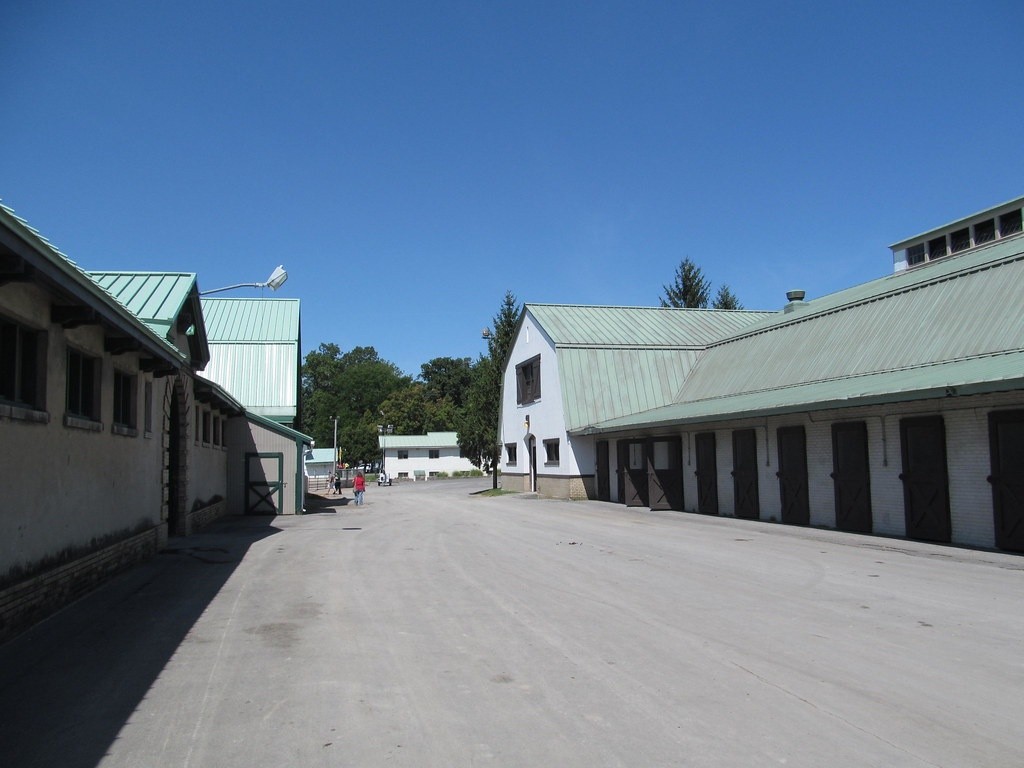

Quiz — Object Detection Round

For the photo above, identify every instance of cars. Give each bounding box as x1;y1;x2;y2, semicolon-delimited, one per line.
354;464;371;473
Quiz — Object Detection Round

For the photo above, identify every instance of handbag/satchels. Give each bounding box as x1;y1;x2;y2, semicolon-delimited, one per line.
353;488;358;493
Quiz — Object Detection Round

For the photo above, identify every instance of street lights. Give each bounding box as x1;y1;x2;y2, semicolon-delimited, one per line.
329;416;341;476
377;425;393;473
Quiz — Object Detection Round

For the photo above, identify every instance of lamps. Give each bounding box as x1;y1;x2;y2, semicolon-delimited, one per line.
199;265;288;296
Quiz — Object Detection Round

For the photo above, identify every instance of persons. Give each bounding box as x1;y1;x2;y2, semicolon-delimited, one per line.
352;472;366;505
326;472;337;494
332;472;342;494
